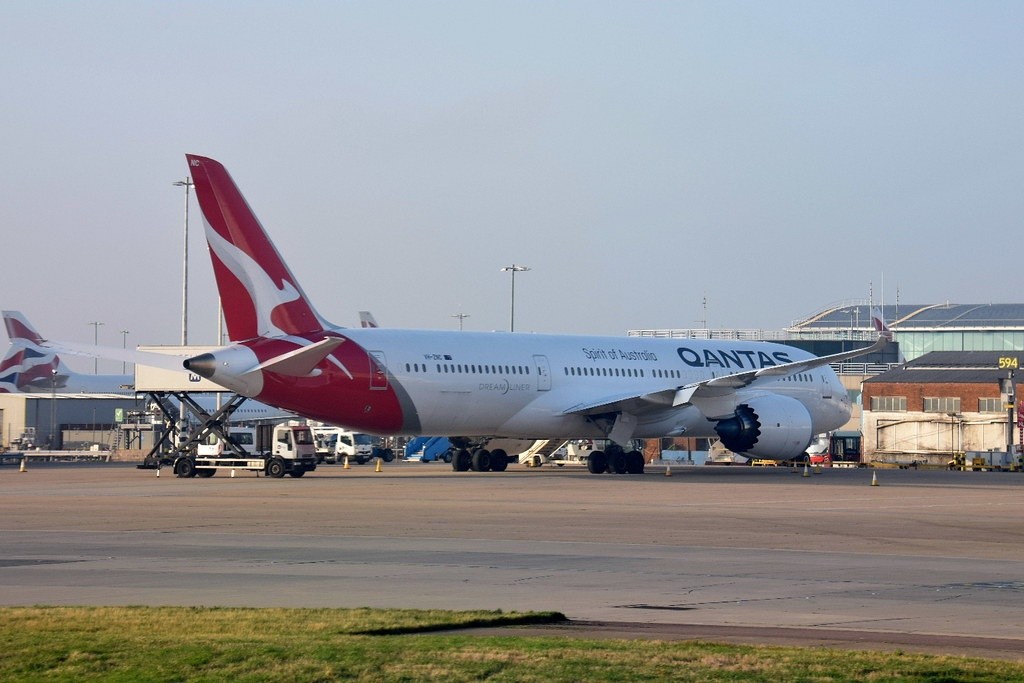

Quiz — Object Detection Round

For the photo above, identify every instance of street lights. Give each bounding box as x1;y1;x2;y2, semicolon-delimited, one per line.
450;313;471;331
169;175;196;459
118;329;128;374
500;263;532;333
90;321;104;375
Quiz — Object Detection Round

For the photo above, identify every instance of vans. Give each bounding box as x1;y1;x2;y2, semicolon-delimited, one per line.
200;427;258;466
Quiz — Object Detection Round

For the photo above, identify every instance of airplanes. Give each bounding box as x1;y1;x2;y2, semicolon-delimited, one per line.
37;151;896;476
0;309;306;458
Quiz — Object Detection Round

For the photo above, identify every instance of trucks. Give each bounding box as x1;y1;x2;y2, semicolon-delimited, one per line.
309;427;374;464
174;425;317;478
